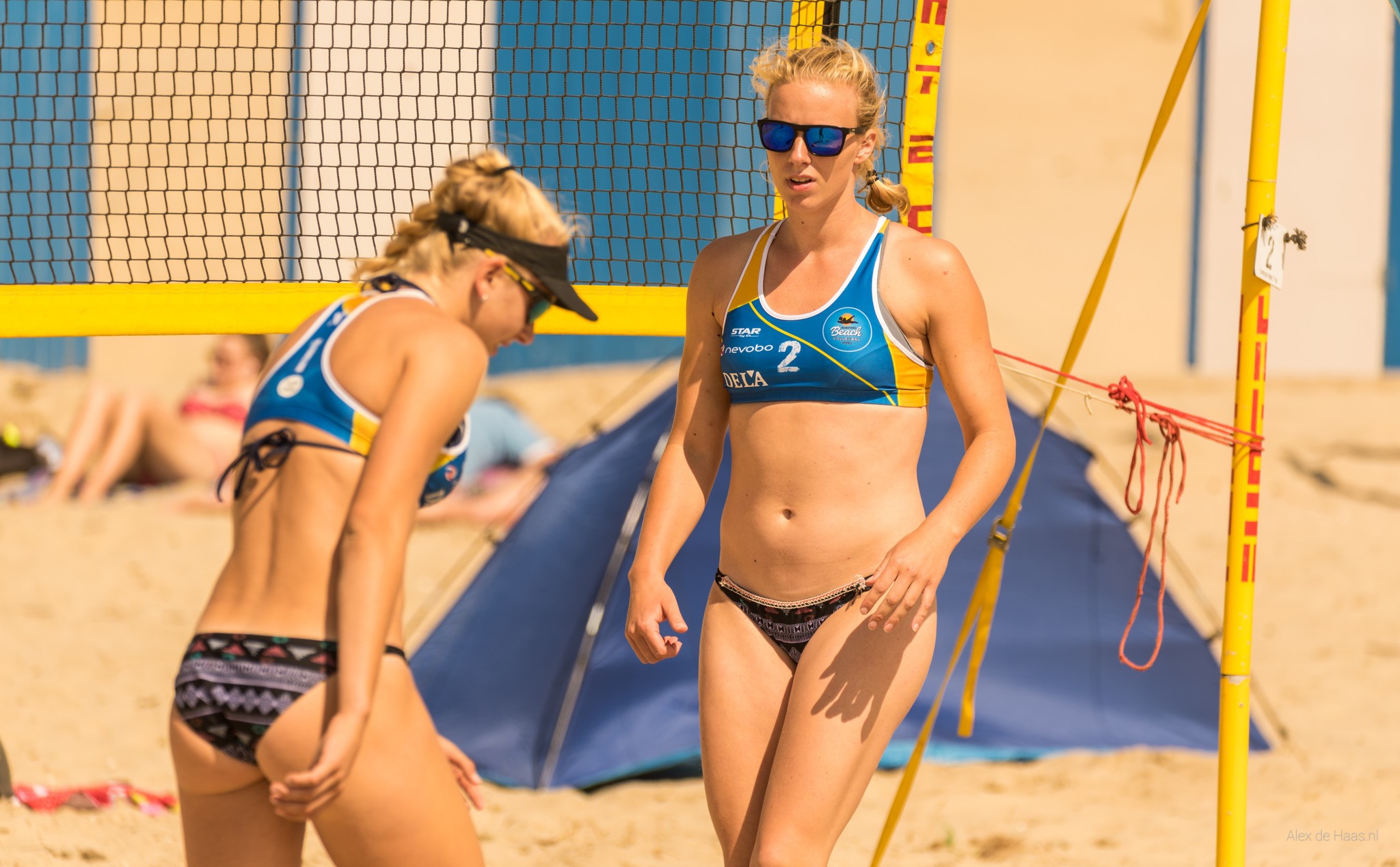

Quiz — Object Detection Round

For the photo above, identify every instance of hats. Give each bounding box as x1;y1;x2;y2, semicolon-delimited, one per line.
433;210;599;328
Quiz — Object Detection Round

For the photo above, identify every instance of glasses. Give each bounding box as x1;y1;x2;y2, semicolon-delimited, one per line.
756;116;865;157
478;248;553;322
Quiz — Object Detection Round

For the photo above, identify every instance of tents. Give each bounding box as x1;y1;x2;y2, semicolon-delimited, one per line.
397;354;1279;796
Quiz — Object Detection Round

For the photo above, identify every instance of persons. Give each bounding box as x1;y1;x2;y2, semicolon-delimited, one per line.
40;334;555;524
168;144;596;867
626;27;1019;867
